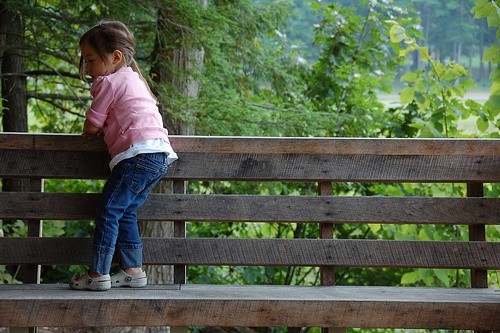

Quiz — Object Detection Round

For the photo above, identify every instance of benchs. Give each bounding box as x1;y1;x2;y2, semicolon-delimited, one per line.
0;132;500;333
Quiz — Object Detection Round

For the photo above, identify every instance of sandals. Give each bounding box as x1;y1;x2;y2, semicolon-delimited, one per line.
70;273;112;290
110;270;148;286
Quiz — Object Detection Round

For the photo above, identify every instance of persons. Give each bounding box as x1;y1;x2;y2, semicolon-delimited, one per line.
69;21;179;291
